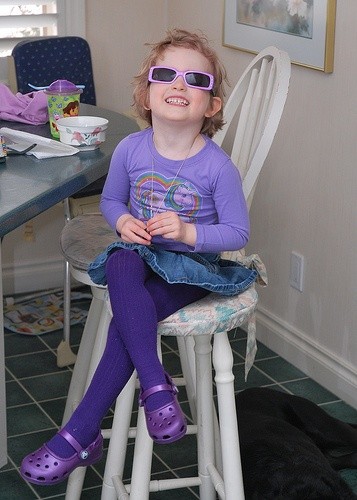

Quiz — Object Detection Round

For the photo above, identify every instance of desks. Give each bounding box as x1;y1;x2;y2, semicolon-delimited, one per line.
0;102;141;469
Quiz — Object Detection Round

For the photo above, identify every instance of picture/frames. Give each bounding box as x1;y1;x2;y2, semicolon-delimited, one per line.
222;0;337;74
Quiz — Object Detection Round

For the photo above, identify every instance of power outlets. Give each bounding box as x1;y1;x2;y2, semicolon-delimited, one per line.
288;251;304;293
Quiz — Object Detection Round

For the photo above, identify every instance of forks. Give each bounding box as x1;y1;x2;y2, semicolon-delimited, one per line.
28;83;85;90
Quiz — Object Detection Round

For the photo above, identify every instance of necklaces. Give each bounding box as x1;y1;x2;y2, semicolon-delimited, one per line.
151;135;199;218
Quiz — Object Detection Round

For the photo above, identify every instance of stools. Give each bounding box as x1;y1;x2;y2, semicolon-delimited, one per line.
98;285;261;500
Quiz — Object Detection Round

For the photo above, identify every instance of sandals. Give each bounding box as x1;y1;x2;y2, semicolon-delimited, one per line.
19;428;105;486
138;371;188;444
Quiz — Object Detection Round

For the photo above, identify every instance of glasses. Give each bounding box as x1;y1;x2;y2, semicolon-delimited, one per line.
148;66;215;91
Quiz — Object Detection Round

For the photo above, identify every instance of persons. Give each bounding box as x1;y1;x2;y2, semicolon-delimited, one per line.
21;28;258;486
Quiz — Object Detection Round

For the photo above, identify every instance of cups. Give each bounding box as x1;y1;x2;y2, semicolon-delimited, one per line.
43;79;83;138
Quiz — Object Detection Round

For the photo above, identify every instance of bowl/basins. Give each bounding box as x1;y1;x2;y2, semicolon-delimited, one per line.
56;116;110;151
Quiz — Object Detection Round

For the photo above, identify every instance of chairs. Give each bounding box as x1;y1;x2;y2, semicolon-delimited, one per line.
14;36;290;500
